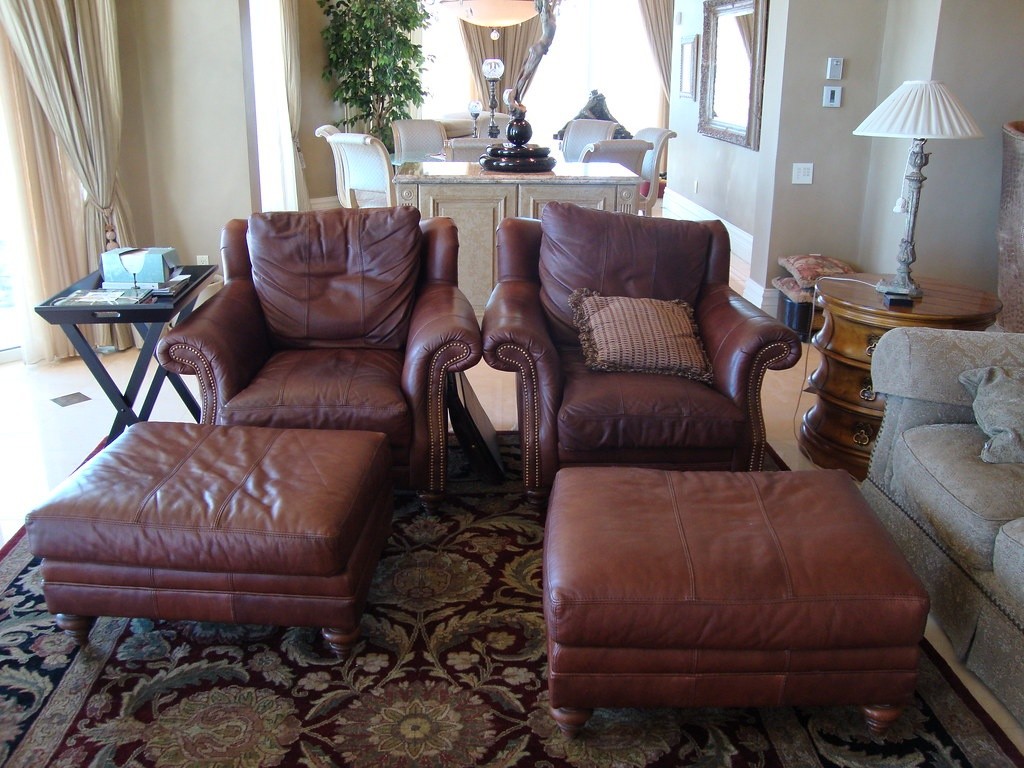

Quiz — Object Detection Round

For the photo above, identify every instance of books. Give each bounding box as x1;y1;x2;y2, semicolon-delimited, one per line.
53;289;158;306
102;274;191;297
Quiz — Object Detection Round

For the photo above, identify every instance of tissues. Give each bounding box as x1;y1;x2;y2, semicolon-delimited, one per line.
100;247;180;283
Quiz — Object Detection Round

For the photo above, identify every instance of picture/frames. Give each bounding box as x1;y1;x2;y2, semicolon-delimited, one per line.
679;34;698;103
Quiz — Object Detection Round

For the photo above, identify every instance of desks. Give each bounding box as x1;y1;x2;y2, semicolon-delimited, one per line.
389;150;445;165
34;264;218;449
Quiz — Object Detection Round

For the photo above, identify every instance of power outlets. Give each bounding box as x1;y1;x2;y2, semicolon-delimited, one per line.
196;255;211;266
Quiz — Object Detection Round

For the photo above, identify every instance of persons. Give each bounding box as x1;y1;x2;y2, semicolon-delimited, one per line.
515;0;558;103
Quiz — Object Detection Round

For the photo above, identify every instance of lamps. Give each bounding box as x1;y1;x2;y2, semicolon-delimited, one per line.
434;0;557;172
852;80;985;300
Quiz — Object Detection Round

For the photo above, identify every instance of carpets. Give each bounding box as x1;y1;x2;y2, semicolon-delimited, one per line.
0;430;1023;768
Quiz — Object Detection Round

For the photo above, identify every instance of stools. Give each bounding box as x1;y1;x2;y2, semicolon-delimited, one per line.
543;467;931;737
25;423;398;661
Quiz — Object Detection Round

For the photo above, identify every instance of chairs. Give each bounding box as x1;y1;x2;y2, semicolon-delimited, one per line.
315;110;676;216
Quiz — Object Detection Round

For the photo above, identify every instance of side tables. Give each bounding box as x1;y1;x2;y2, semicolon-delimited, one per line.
797;272;1004;481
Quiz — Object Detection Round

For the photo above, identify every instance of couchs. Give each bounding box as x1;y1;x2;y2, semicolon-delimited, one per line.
479;201;803;507
156;205;482;515
860;325;1024;728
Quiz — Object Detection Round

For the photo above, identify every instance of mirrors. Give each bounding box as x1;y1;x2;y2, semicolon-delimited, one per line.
696;0;769;153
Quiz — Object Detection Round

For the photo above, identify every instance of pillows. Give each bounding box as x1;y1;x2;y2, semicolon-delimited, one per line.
772;254;857;330
567;286;714;386
957;366;1024;464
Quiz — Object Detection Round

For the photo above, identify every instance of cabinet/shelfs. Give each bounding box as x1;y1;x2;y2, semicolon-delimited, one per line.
391;162;646;317
